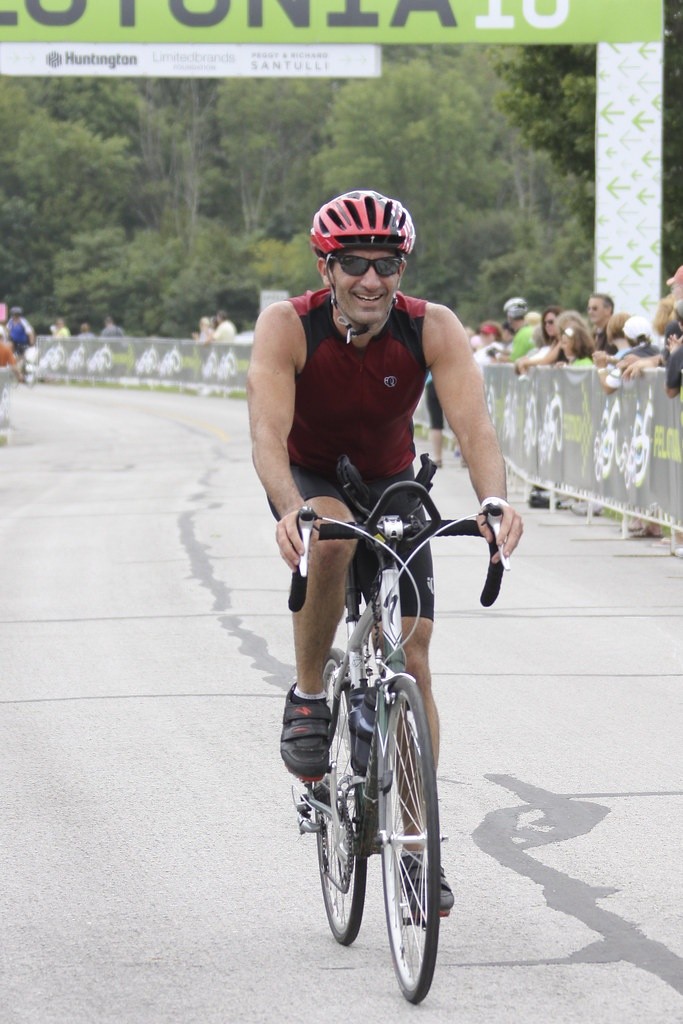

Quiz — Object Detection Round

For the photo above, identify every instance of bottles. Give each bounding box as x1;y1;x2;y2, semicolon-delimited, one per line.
515;359;530;383
486;347;501;359
348;679;382;777
592;350;621;395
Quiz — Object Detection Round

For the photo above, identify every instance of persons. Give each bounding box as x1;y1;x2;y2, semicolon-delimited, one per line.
100;316;128;338
0;327;24;447
425;265;683;559
246;190;523;913
50;318;71;338
190;310;237;397
5;306;35;358
76;323;96;338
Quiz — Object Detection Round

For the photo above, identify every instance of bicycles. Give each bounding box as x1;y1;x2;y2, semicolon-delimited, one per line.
287;482;510;1005
9;343;37;389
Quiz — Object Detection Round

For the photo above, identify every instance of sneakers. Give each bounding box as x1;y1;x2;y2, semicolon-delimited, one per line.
280;682;333;781
400;854;455;917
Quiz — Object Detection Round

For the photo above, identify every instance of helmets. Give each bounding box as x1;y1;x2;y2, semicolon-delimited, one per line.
310;190;417;258
503;297;529;317
10;307;23;315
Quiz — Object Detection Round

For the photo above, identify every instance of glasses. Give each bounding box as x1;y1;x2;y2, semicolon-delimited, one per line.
324;254;404;276
543;319;554;326
587;305;606;311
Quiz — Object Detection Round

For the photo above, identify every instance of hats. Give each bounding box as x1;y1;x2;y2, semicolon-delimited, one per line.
480;325;498;334
622;315;654;342
666;265;683;286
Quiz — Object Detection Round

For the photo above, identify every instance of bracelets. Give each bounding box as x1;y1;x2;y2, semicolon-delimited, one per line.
598;368;608;376
